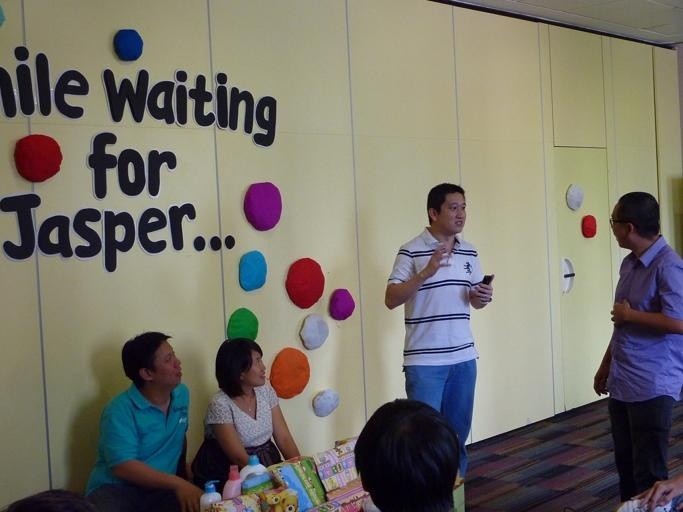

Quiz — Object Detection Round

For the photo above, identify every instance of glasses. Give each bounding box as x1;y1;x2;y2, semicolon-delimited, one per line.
610;217;625;226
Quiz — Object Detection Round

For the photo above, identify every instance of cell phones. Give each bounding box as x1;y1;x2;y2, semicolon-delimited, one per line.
481;274;492;284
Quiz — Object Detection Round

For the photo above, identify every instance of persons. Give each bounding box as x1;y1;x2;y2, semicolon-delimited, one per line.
79;326;206;512
384;182;493;493
189;333;304;497
590;192;682;504
354;395;462;510
629;475;683;511
